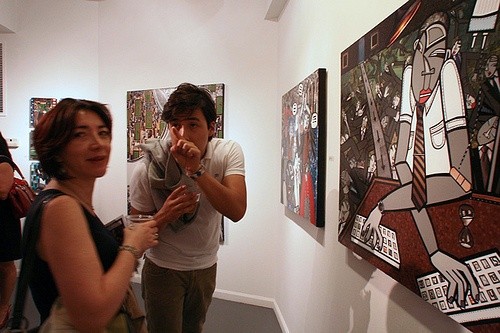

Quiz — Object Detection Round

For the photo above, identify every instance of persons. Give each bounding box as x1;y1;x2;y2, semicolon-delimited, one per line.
20;98;160;333
0;133;24;332
130;82;247;333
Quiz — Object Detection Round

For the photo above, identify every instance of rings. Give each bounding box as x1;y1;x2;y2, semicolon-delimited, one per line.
155;234;158;238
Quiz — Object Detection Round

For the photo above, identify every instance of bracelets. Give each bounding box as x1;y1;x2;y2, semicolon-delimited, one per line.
190;166;206;180
119;245;141;258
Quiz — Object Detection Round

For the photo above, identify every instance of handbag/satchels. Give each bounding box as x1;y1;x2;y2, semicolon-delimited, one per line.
0;156;37;219
4;191;149;333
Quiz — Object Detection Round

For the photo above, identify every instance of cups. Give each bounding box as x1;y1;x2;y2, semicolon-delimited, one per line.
183;192;202;214
125;214;153;223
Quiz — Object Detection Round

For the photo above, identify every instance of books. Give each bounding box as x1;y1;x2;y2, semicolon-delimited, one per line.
105;214;128;240
